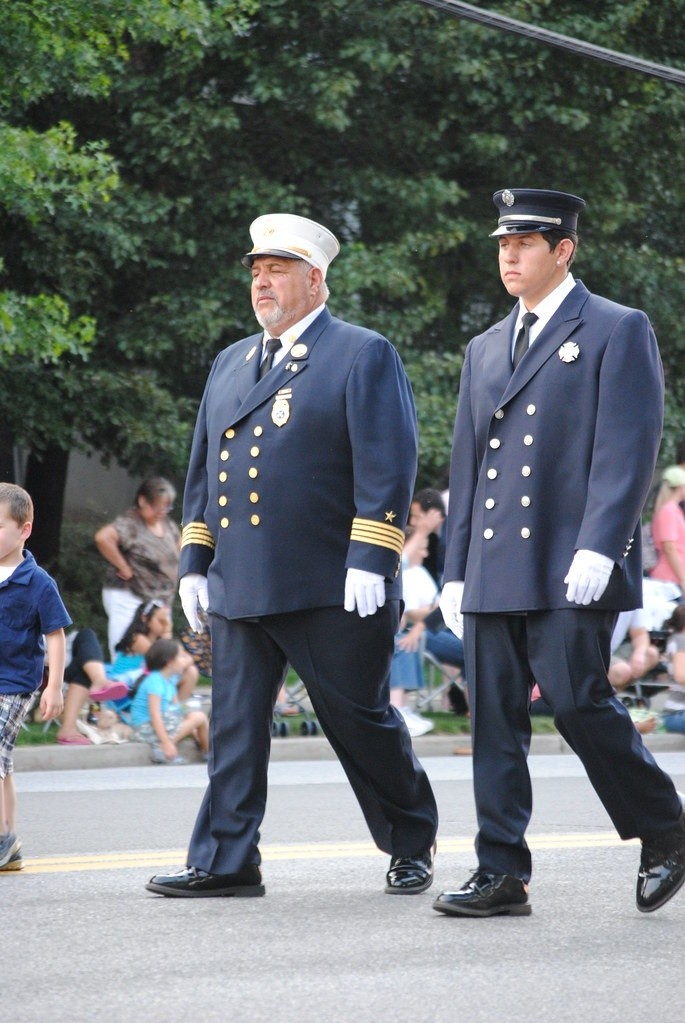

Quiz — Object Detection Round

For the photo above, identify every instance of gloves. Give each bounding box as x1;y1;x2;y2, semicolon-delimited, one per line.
343;568;386;617
564;550;616;606
179;574;209;635
440;580;466;641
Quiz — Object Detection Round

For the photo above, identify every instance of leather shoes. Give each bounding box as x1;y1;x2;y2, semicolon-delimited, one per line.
384;838;436;896
636;811;685;914
145;860;265;897
432;867;532;916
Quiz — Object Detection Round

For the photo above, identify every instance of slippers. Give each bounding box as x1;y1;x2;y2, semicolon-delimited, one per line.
58;733;89;746
89;682;129;701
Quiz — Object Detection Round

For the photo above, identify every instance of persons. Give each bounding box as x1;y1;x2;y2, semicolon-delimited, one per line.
606;459;685;734
273;684;304;718
42;476;211;766
432;190;685;916
141;214;438;898
0;482;61;871
381;487;470;737
528;683;554;716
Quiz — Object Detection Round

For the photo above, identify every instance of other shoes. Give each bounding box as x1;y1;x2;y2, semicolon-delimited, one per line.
202;752;210;761
150;747;187;765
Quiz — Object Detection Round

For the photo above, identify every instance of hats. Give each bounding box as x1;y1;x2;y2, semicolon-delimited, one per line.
488;189;584;236
245;214;341;278
661;465;685;489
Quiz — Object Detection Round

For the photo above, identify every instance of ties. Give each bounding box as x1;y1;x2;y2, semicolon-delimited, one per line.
258;339;282;381
511;313;538;369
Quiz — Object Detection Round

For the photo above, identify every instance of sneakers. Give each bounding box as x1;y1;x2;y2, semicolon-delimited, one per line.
0;835;20;867
400;707;435;736
1;852;23;870
